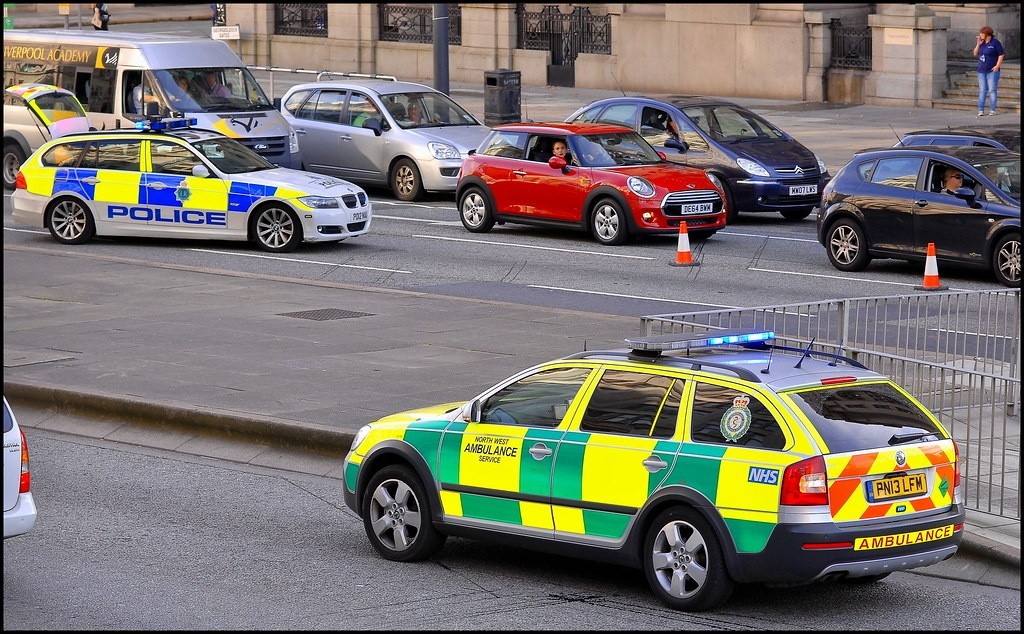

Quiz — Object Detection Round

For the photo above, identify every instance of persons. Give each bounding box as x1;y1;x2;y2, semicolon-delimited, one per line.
133;71;232;114
552;139;570;163
668;116;678;139
91;3;111;31
352;98;387;127
936;166;964;194
974;26;1004;116
973;166;1011;198
404;103;426;123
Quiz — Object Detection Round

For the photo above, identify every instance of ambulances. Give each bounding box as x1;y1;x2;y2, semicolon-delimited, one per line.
340;329;969;612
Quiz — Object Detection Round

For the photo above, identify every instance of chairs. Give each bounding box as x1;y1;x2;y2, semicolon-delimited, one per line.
928;164;946;195
387;103;406;123
190;75;202;98
644;111;666;131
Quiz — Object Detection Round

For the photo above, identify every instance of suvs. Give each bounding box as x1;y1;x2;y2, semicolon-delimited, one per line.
563;96;833;223
278;80;513;201
3;395;38;540
891;125;1024;177
817;145;1024;288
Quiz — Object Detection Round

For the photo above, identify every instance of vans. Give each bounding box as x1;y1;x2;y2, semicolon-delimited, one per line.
3;30;302;190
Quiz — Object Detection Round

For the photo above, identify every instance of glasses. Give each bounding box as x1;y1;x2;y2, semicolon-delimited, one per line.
950;174;964;179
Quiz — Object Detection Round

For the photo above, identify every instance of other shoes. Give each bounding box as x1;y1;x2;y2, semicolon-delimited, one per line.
978;111;984;116
989;111;995;116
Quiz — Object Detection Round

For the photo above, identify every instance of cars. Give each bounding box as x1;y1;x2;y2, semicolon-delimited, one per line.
4;82;373;254
455;120;727;245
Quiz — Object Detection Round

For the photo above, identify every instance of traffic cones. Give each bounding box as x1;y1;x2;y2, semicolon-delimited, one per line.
668;220;702;268
914;243;950;291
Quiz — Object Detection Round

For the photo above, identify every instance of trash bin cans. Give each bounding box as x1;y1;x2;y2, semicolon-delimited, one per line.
482;68;522;128
